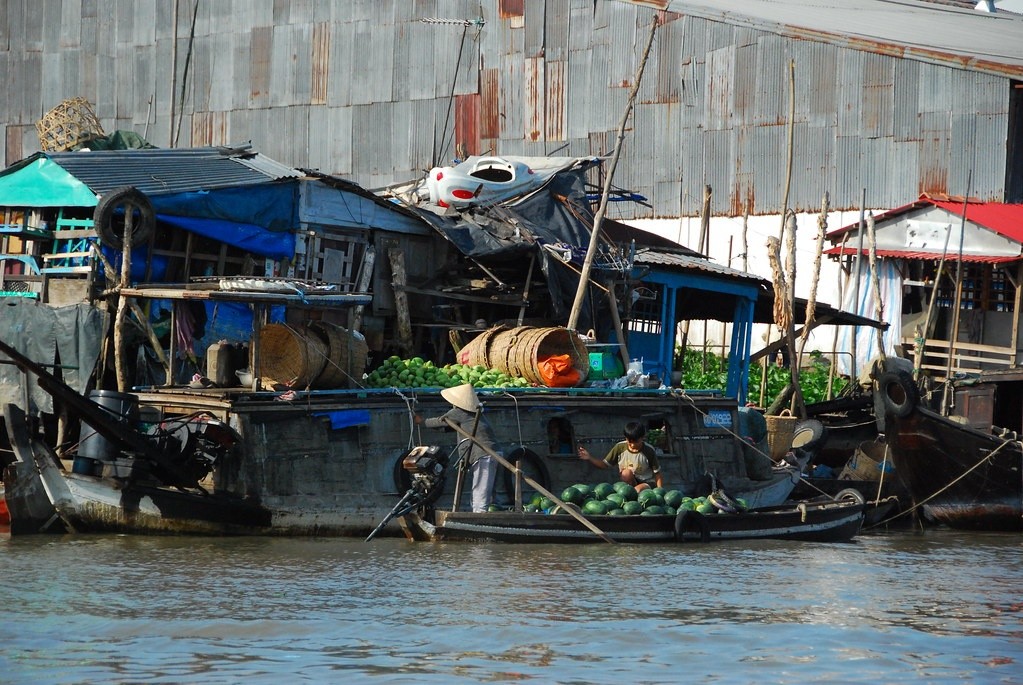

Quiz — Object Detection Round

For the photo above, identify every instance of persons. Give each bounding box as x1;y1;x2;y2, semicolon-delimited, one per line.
578;421;664;492
413;383;514;513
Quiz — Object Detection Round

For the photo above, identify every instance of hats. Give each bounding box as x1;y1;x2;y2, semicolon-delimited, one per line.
440;382;485;412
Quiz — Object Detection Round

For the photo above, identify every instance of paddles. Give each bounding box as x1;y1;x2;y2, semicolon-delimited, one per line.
451;401;488;512
439;414;617;544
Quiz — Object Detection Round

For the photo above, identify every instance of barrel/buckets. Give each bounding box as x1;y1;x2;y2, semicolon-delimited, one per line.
206;340;235;386
78;392;139;461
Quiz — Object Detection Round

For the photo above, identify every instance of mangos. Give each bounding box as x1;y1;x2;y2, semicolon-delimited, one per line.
365;355;532;390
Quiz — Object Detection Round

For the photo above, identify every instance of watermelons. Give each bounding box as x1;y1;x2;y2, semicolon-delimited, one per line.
488;482;748;516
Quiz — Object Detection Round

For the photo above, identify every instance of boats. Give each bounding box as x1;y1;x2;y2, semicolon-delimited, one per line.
881;370;1022;535
1;363;908;543
362;441;877;547
